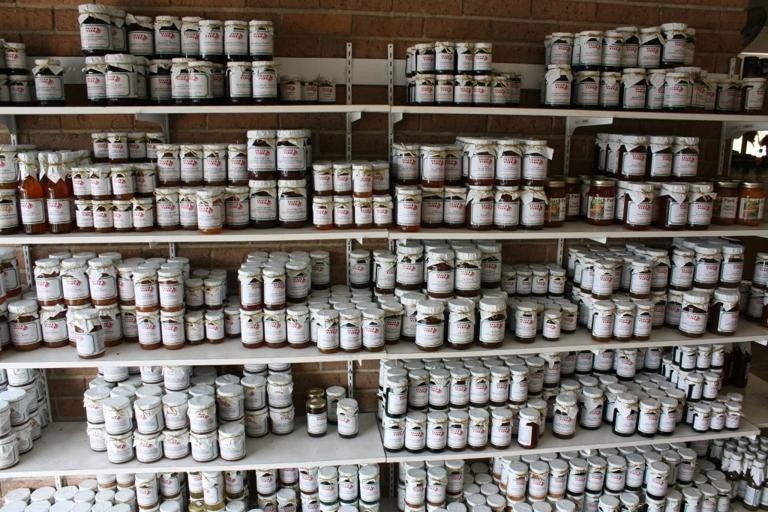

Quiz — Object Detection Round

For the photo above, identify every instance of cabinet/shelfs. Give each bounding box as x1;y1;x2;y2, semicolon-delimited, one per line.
0;41;768;511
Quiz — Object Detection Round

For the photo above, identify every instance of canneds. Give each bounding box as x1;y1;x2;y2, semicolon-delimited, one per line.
1;129;765;234
84;364;296;463
375;344;751;452
0;464;381;512
324;385;347;423
0;3;768;115
336;399;359;439
0;368;50;470
306;397;327;437
398;435;768;512
0;237;768;358
305;388;325;400
